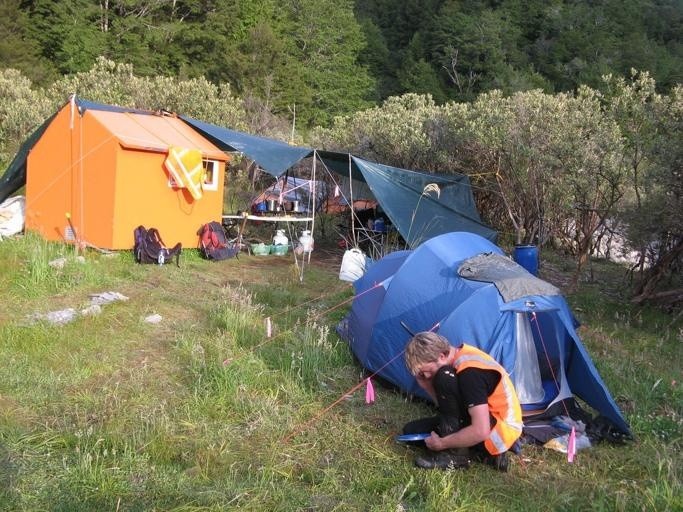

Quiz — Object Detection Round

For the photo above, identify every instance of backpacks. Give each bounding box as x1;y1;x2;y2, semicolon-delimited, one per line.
197;218;239;260
131;225;183;264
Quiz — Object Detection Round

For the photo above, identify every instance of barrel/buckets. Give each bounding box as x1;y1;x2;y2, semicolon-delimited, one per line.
514;243;539;275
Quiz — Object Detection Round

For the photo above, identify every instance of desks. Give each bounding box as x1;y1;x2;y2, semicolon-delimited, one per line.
353;227;397;259
222;214;312;251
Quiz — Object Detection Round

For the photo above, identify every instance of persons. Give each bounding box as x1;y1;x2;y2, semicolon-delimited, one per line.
403;332;523;470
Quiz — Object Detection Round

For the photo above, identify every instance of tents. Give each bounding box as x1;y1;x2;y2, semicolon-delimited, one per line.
337;232;631;435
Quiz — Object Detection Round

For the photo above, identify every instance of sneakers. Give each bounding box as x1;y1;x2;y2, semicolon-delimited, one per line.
416;451;471;469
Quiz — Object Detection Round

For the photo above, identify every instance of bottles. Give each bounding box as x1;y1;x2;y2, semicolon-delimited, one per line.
158;250;165;264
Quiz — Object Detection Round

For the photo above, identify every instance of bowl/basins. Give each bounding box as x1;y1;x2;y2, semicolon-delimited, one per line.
269;245;292;256
251;243;271;257
